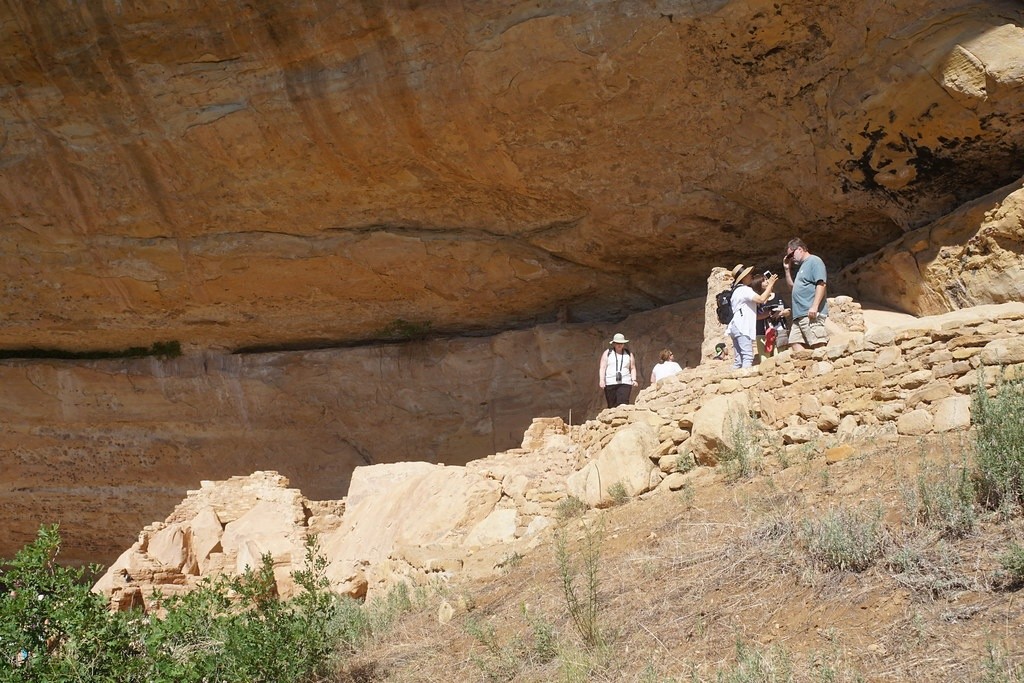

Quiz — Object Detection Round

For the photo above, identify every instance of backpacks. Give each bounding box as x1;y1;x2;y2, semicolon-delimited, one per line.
716;284;743;325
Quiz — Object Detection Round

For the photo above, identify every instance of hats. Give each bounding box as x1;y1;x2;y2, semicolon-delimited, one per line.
715;343;726;357
609;333;630;345
732;263;755;288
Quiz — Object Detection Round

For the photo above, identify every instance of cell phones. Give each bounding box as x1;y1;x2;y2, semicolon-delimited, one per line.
764;270;773;279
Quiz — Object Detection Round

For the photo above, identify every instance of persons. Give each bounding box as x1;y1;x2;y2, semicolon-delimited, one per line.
753;274;790;356
724;263;778;370
650;349;682;386
782;238;828;352
599;333;639;409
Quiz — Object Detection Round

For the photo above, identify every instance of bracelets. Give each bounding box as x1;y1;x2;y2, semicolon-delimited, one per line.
632;380;637;382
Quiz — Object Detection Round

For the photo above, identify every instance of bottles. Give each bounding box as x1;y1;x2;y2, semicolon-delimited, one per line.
778;299;784;310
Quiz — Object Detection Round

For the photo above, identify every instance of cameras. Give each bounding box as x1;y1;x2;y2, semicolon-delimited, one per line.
616;372;621;381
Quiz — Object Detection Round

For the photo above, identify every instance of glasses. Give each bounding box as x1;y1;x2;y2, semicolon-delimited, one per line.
669;353;674;357
786;249;797;260
